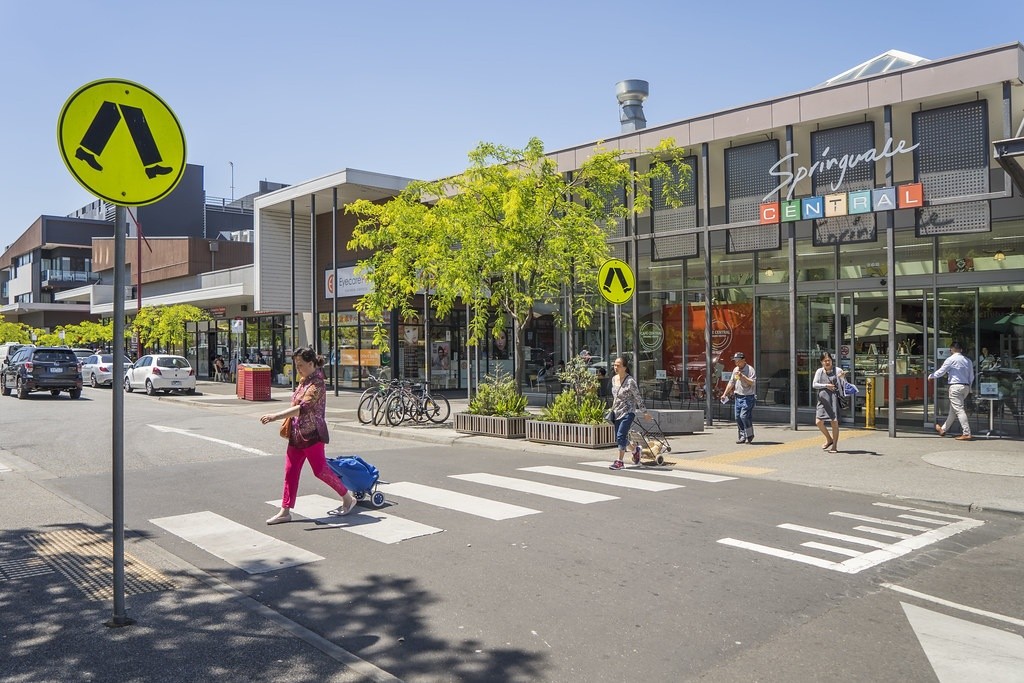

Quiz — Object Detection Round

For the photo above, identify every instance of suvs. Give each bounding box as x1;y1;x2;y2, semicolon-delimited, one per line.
0;342;37;369
590;352;658;381
0;345;84;399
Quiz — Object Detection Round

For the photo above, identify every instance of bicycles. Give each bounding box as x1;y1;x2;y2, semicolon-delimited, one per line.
356;366;451;427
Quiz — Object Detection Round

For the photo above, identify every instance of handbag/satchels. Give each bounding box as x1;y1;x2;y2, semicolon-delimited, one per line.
280;416;291;438
605;411;615;425
838;397;847;408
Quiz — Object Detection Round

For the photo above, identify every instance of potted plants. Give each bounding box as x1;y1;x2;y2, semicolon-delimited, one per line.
524;354;616;449
451;358;533;439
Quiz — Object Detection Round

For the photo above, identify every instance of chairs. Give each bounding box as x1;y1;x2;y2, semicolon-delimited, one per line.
544;375;564;407
597;378;612;409
651;380;699;410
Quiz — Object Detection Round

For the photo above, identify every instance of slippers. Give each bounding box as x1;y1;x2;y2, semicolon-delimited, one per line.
828;449;837;453
823;442;833;449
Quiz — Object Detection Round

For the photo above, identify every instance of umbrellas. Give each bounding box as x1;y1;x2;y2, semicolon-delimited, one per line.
961;311;1024;337
843;316;951;355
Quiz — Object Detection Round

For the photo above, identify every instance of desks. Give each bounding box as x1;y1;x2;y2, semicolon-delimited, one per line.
977;397;1010;439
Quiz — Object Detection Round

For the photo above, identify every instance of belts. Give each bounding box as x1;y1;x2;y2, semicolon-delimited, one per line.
951;383;970;386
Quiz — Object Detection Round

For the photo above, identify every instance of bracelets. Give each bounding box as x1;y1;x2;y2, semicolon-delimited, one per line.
644;413;648;415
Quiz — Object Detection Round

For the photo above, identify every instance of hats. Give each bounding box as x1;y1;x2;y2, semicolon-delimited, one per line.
732;353;745;361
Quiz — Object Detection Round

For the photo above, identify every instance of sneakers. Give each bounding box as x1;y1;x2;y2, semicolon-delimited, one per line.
632;445;642;466
609;460;625;470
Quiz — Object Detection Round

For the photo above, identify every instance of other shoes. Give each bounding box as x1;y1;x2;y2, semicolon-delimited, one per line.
935;424;946;436
737;439;745;444
955;435;972;441
267;513;291;524
748;435;754;442
339;497;357;516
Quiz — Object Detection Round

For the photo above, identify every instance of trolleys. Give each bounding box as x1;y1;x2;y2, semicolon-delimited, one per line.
625;421;672;466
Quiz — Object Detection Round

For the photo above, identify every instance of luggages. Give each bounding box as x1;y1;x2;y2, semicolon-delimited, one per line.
325;454;390;507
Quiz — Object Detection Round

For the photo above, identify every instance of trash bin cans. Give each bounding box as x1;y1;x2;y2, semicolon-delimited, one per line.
236;363;272;400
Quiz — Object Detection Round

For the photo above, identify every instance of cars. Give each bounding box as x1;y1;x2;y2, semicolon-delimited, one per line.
566;355;603;375
80;354;134;388
72;349;96;366
124;354;197;395
675;349;733;380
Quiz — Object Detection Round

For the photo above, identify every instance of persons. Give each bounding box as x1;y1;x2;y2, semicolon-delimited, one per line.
721;352;757;444
260;346;357;524
697;368;719;399
581;332;601;356
546;345;652;470
402;314;419;346
493;329;510;361
928;343;999;440
813;351;847;454
433;346;449;370
213;350;267;383
